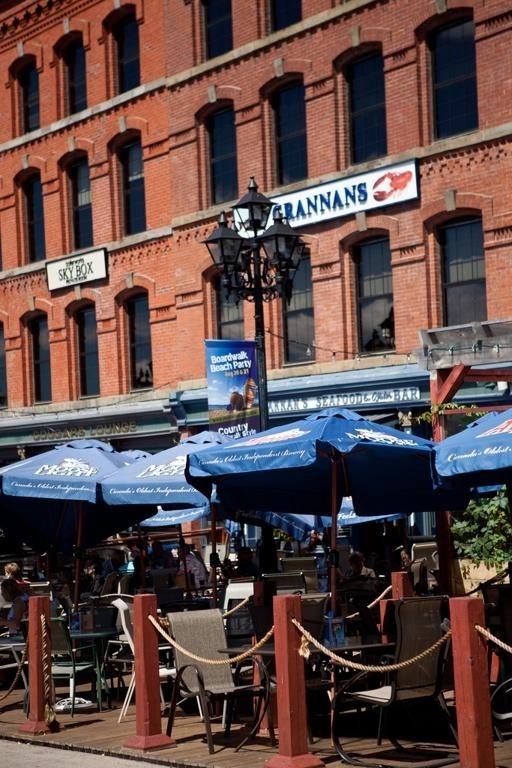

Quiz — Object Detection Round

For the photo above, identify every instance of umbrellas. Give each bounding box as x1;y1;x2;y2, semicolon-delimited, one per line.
99;430;235;609
184;405;436;616
1;436;140;611
435;402;512;488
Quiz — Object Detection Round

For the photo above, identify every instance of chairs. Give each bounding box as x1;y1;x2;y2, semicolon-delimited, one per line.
165;609;274;754
338;595;458;756
2;543;164;726
480;584;512;742
168;544;452;604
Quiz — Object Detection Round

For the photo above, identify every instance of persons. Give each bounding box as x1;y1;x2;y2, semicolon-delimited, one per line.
0;537;419;650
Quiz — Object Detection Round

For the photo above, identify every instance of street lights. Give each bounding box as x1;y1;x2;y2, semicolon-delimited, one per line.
200;177;312;431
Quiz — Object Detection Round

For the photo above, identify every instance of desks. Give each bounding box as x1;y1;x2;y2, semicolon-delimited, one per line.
218;638;397;755
